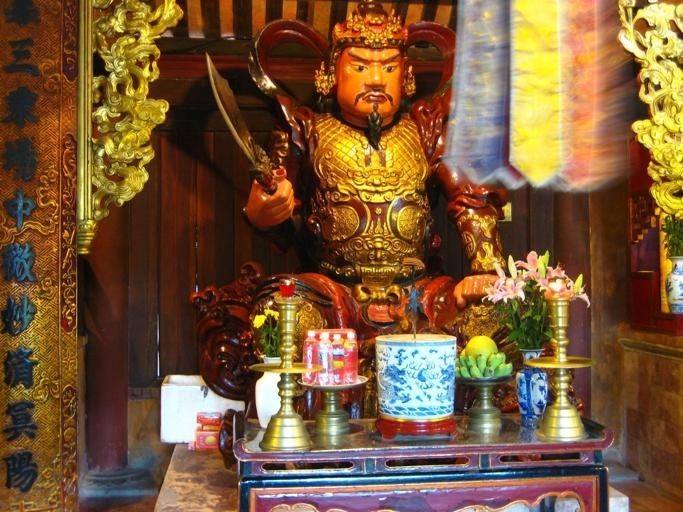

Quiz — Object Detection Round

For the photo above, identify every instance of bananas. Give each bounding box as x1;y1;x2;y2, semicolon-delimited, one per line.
455;352;513;378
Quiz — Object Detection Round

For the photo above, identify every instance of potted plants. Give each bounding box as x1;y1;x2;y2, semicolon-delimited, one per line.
660;209;683;313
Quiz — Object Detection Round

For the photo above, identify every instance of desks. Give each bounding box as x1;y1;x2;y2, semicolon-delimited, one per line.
232;415;615;511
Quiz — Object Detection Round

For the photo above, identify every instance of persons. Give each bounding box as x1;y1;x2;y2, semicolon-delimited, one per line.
239;0;585;413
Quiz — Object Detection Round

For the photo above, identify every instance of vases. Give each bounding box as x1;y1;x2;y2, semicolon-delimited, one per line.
375;334;456;419
255;355;281;429
516;349;548;431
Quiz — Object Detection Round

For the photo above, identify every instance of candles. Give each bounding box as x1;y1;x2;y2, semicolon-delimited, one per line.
279;279;296;297
549;279;569;299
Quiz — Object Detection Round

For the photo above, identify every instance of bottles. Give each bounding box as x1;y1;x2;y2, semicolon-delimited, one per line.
302;329;359;387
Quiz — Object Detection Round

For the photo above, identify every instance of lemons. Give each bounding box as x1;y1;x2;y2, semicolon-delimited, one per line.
466;336;499;358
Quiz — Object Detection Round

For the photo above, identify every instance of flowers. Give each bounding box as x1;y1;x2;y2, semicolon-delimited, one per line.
405;286;425;340
481;249;591;350
253;309;299;358
